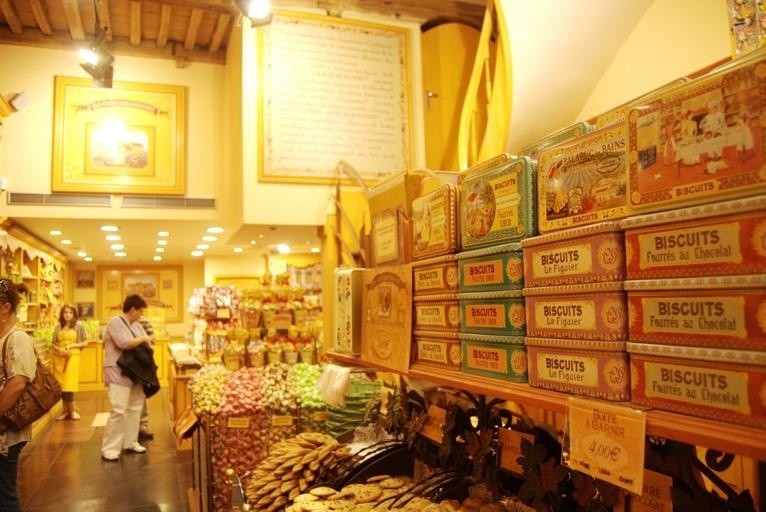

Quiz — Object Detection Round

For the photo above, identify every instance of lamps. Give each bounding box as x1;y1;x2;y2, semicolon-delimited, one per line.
237;0;271;22
80;28;115;90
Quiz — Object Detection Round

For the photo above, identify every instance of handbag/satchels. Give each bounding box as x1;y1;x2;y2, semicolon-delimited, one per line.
0;329;61;432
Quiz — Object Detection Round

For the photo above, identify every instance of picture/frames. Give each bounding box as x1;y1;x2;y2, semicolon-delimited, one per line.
254;9;413;189
75;302;95;319
96;261;184;327
50;71;187;197
76;270;95;289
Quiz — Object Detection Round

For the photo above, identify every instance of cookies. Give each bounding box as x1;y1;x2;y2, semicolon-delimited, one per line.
285;474;520;512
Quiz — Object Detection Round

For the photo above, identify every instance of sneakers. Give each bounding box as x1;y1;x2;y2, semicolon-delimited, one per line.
128;445;146;453
102;454;118;460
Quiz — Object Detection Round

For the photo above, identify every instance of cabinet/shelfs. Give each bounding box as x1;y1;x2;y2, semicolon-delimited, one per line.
2;226;70;443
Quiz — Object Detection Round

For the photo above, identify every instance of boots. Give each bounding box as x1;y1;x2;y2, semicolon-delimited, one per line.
67;401;79;420
55;401;69;421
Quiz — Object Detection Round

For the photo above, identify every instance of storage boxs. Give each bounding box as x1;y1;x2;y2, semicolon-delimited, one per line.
333;44;766;433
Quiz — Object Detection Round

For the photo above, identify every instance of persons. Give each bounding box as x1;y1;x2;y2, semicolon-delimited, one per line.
50;304;89;422
0;277;39;511
135;315;156;439
98;293;157;462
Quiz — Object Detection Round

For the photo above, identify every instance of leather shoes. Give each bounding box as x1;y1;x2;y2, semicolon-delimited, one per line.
139;429;153;438
